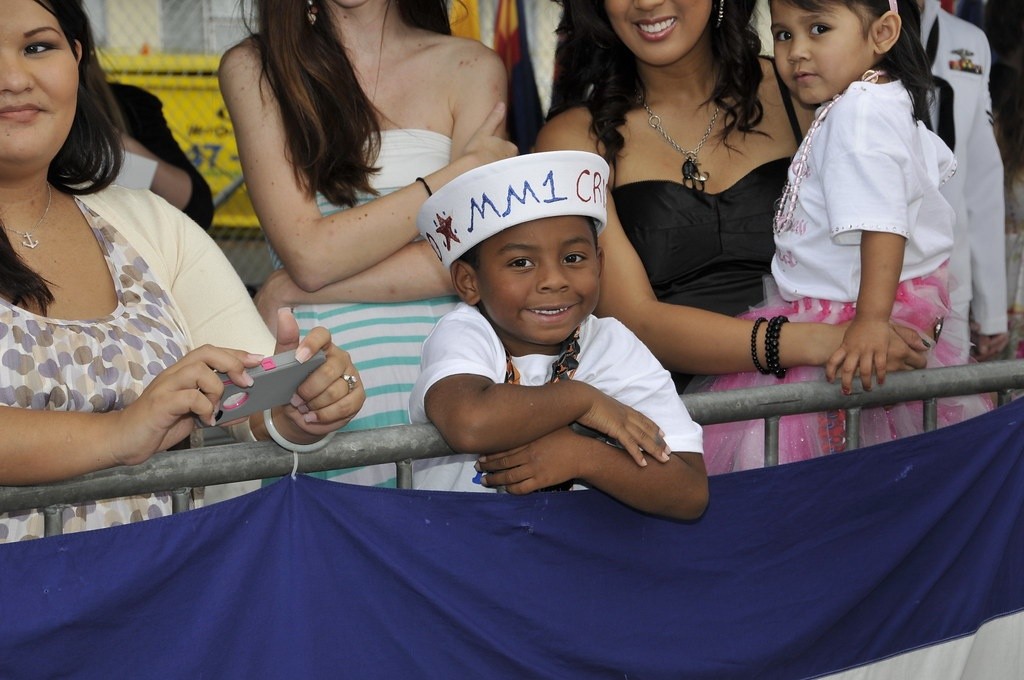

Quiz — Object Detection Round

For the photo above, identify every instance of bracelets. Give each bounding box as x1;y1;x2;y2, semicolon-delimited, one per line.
416;177;433;196
263;409;339;454
933;319;943;342
751;315;789;378
229;417;257;444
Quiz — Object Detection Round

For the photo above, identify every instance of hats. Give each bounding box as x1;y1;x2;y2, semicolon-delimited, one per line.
416;150;610;270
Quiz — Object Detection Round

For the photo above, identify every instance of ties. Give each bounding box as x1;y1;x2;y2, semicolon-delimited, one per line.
921;14;956;150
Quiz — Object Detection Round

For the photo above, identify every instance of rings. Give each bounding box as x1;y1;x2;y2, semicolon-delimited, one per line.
342;374;357;393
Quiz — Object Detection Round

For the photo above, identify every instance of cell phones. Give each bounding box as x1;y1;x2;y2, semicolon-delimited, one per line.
194;347;326;428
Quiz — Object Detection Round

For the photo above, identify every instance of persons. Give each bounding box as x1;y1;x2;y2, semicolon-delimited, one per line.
682;0;996;476
0;0;364;542
915;0;1010;357
217;0;521;493
531;0;930;397
407;150;709;521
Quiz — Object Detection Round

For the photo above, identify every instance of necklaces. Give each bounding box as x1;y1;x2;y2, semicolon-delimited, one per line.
503;324;580;386
773;68;885;234
635;93;719;180
2;181;52;249
